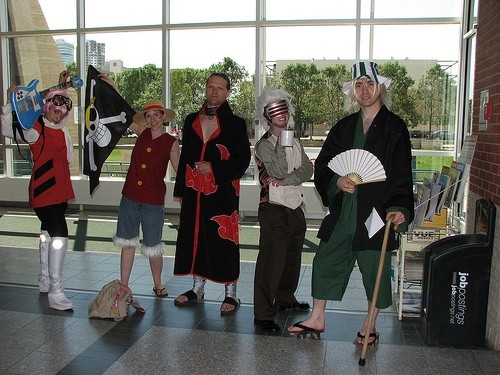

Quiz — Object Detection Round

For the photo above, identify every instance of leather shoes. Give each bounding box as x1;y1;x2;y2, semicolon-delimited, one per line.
254;318;280;332
274;300;310;311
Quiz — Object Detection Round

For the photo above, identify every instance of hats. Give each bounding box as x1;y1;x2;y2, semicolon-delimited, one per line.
342;62;391;96
132;100;176;126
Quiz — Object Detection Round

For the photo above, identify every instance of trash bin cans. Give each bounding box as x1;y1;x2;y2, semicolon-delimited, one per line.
421;199;496;346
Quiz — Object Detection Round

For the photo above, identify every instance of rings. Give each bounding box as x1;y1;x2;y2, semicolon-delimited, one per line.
195;165;198;168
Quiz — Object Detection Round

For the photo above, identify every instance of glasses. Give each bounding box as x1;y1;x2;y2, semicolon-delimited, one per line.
52;95;72;110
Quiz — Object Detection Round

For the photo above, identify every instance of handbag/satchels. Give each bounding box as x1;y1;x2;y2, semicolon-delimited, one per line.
87;279;146;319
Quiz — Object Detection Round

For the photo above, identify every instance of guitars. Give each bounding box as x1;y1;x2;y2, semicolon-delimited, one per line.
9;75;83;132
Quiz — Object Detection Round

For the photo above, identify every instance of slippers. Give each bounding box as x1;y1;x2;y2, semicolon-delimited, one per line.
358;332;379;345
173;290;204;305
153;287;168;296
220;296;240;315
288;321;324;334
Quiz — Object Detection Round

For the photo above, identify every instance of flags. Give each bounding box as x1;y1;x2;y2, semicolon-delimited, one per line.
82;65;137;199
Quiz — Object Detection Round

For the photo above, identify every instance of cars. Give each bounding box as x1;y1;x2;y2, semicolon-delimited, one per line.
431;130;456;140
425;131;434;137
325;131;329;135
411;131;423;138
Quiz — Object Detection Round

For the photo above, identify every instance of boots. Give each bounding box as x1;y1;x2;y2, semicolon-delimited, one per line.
38;230;50;293
48;237;72;310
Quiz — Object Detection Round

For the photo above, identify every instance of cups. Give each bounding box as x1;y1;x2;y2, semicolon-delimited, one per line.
280;130;294;147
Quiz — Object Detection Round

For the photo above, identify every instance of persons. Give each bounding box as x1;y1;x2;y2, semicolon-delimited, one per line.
173;73;251;313
0;70;75;310
288;62;414;347
254;88;313;321
97;73;181;297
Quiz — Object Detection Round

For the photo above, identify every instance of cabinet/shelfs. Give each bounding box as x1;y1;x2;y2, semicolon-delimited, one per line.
396;231;455;318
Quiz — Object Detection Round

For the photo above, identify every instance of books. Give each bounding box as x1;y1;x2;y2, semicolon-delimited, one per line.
407;161;469;232
